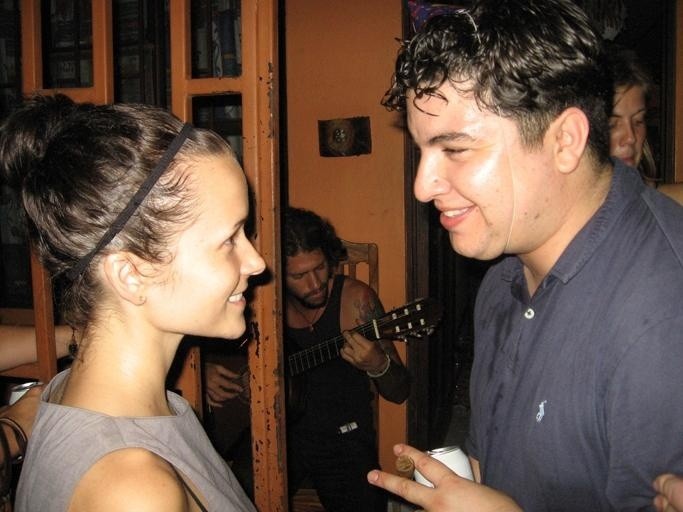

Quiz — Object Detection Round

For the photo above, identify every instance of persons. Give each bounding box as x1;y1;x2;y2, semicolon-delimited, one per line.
1;92;266;512
365;4;683;512
648;471;682;511
0;315;86;512
604;59;683;208
198;203;412;512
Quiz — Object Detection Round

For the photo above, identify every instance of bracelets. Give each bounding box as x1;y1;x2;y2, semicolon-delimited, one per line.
364;352;392;377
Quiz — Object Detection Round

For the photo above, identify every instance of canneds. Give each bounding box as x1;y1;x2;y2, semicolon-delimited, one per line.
413;444;475;488
8;381;44;406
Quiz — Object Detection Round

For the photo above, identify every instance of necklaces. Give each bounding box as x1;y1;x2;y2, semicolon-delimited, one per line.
286;297;320;331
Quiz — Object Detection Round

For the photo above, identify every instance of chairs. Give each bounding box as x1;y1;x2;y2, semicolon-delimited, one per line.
282;234;401;511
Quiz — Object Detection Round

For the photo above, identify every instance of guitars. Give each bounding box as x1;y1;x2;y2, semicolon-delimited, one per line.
201;295;445;454
